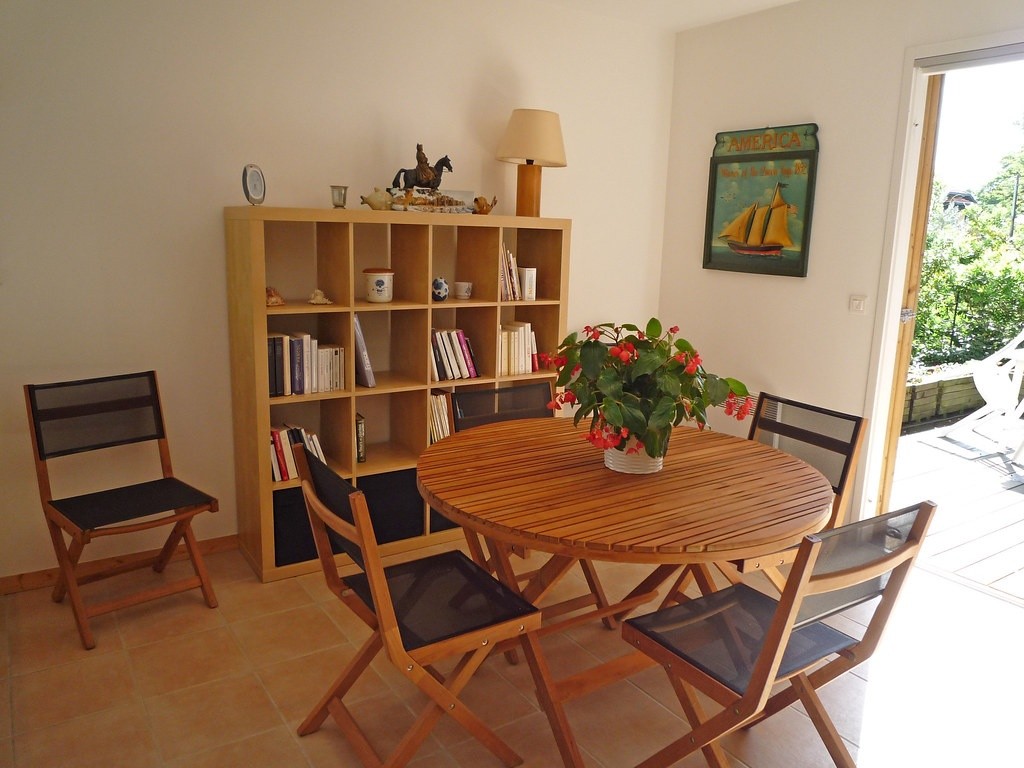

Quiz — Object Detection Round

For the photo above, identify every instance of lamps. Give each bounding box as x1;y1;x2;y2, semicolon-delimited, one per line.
496;108;568;217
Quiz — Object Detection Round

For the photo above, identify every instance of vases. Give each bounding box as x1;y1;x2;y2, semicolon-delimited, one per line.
603;424;664;474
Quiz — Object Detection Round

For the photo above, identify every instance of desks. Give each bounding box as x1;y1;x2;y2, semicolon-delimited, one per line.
416;417;835;768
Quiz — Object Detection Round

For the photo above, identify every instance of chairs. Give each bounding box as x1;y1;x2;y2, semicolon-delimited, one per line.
22;369;221;649
621;498;938;768
445;380;618;665
660;392;869;611
288;442;543;768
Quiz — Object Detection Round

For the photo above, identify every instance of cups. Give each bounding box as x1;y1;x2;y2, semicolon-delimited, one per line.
330;184;349;209
363;269;394;302
455;281;473;299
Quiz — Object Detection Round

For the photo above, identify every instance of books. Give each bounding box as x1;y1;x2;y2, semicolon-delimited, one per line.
501;242;538;303
266;328;345;397
498;321;540;377
429;328;482;381
355;412;367;465
270;422;328;483
429;389;464;446
354;313;377;388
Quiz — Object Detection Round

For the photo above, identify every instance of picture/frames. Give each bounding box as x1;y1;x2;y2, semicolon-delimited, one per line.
702;149;820;279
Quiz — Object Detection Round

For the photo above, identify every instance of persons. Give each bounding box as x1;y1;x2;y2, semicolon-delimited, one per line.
415;143;436;184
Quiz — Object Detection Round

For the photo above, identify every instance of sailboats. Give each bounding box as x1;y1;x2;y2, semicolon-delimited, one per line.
716;180;794;256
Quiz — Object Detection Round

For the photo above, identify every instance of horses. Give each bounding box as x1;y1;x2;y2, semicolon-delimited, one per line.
392;155;452;192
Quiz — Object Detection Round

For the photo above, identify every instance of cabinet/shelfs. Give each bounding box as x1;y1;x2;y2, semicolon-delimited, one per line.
222;206;572;585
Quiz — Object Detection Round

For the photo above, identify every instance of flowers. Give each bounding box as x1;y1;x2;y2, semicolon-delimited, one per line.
537;317;754;458
